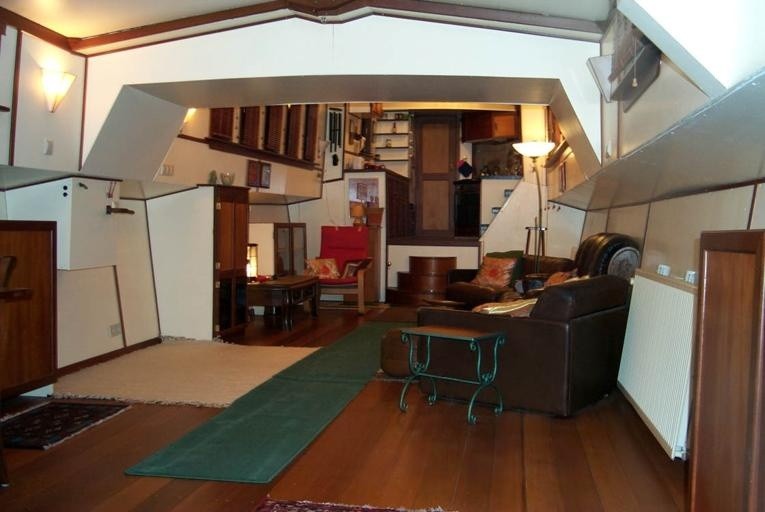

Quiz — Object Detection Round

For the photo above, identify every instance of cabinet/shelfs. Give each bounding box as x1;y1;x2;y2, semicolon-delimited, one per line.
461;112;519;143
343;169;413;239
213;185;249;335
478;175;521;236
373;109;415;162
1;220;57;399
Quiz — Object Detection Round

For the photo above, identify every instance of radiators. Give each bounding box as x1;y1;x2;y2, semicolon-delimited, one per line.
616;267;699;461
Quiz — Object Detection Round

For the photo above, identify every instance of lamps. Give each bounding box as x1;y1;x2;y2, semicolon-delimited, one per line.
42;67;77;114
512;141;556;273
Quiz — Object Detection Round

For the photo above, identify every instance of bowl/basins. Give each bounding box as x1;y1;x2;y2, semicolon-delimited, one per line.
395;114;404;120
220;172;235;185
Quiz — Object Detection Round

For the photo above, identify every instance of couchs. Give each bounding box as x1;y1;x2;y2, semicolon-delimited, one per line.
424;231;641;420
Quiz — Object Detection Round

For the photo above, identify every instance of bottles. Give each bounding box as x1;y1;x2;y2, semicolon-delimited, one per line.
392;122;396;133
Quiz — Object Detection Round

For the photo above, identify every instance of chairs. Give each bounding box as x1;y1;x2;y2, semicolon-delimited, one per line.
303;225;376;316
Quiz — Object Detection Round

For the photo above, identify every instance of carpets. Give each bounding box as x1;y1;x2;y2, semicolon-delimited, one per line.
123;322;417;485
249;492;441;511
0;400;133;451
52;338;325;408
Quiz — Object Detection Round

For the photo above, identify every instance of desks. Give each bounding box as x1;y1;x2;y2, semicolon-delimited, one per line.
399;324;507;425
248;276;321;332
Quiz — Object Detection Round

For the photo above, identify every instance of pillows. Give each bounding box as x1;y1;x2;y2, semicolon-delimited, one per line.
341;257;370;279
304;256;341;279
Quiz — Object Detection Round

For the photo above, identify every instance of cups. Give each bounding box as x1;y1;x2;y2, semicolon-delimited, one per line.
375;154;380;159
365;162;385;170
657;264;671;276
352;157;364;170
685;271;696;284
386;139;391;147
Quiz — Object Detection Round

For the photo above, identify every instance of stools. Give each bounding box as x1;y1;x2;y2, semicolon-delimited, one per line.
381;327;420;378
